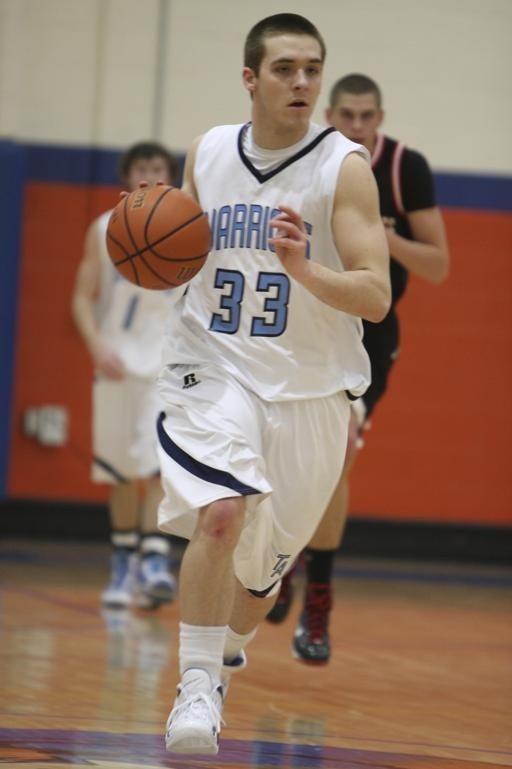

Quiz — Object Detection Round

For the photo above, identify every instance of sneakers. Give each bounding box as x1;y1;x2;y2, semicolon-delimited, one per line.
164;646;249;756
97;548;175;610
263;570;335;667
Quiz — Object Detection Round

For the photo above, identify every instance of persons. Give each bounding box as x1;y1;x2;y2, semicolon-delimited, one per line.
69;137;179;614
258;69;452;672
118;9;400;762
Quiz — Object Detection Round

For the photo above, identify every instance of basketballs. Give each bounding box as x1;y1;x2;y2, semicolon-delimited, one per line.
106;185;211;291
